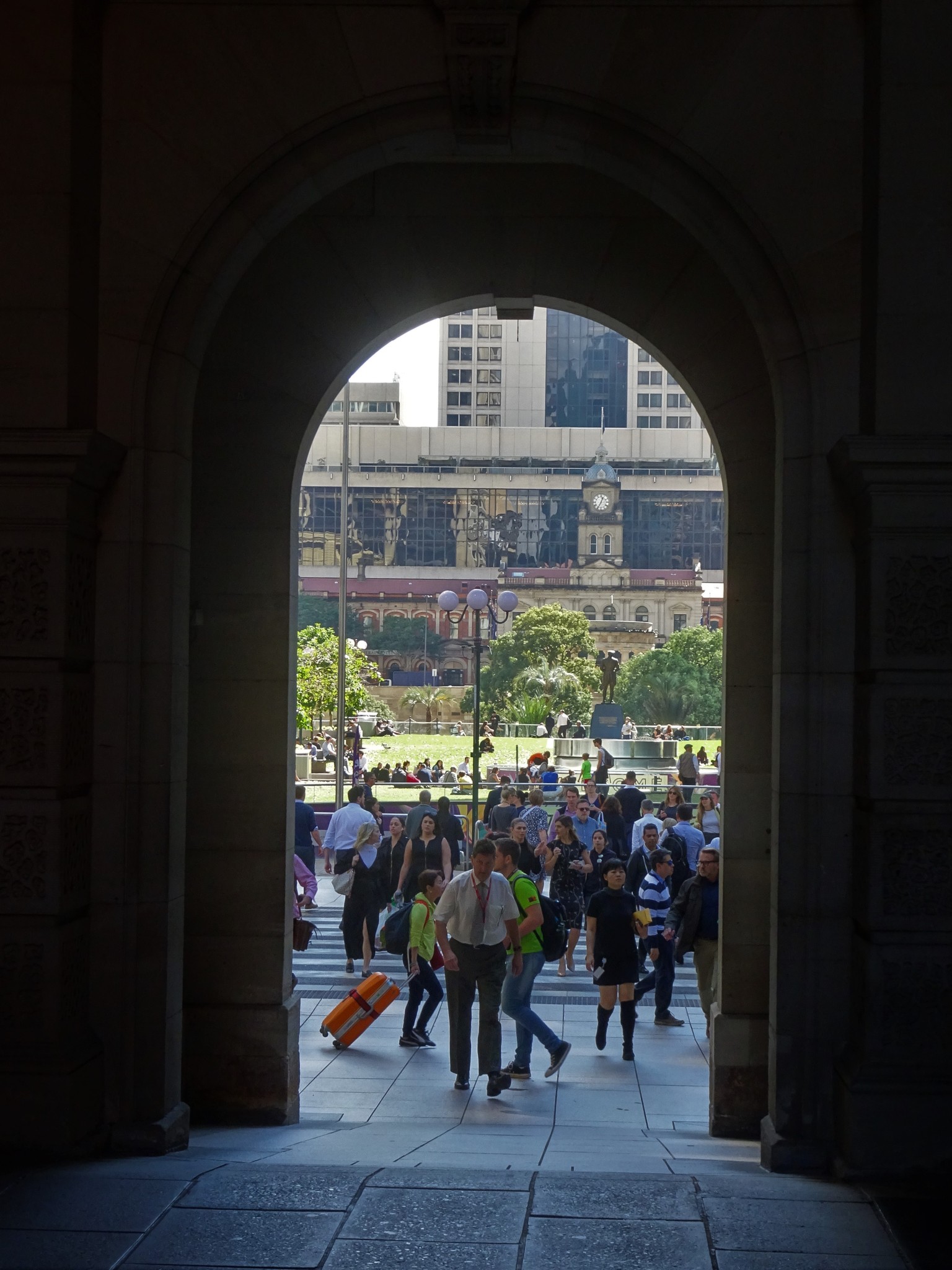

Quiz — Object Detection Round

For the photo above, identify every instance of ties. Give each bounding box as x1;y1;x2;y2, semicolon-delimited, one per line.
470;882;485;948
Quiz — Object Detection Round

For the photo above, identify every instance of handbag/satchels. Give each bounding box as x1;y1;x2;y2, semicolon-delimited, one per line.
714;753;720;768
597;811;607;832
567;718;572;729
293;918;317;952
331;847;404;950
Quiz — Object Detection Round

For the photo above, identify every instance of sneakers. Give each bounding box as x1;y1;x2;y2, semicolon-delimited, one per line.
500;1040;572;1078
399;1028;436;1047
654;1010;685;1026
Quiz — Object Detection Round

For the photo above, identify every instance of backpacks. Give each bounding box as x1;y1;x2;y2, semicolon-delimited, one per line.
513;875;568;962
600;748;614;769
661;826;692;881
379;903;413;956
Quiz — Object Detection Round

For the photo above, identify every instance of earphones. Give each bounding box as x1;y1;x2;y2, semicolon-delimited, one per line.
401;828;403;831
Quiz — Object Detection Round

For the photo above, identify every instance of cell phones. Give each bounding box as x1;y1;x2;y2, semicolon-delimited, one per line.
631;918;643;930
590;804;594;807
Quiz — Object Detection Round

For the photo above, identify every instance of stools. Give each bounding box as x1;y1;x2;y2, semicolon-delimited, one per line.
311;760;326;773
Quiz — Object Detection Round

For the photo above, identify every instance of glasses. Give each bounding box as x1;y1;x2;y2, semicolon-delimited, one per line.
371;778;376;780
669;791;676;795
662;859;719;867
578;807;590;812
588;785;595;787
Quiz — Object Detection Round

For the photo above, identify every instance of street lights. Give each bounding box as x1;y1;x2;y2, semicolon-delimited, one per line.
437;590;519;848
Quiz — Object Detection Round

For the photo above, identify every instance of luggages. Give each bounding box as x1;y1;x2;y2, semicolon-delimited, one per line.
320;970;418;1050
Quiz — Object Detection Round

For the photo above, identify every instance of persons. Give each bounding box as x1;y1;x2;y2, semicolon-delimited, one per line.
291;709;722;1099
600;650;620;704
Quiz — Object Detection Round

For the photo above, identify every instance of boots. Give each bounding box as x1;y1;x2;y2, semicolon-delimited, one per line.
595;999;636;1061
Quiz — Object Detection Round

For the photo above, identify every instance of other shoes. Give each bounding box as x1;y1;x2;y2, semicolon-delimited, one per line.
705;1015;710;1038
305;903;318;909
346;960;373;978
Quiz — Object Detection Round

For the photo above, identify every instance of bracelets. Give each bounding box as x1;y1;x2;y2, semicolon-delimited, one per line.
513;946;522;951
409;962;418;967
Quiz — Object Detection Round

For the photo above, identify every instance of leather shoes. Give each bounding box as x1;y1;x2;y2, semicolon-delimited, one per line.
454;1072;511;1097
639;964;649;973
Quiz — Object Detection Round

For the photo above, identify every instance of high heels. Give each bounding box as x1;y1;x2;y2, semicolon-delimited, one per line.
557;954;575;977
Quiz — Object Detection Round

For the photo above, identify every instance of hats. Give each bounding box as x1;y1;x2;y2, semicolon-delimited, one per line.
700;788;720;799
684;744;693;749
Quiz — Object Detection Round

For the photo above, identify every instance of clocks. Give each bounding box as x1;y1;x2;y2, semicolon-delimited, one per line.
593;494;609;511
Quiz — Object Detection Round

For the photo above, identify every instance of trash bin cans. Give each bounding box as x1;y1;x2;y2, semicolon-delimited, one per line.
295;749;311;778
357;712;378;722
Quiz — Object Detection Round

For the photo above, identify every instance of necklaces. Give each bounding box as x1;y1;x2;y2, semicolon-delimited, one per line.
503;801;509;804
